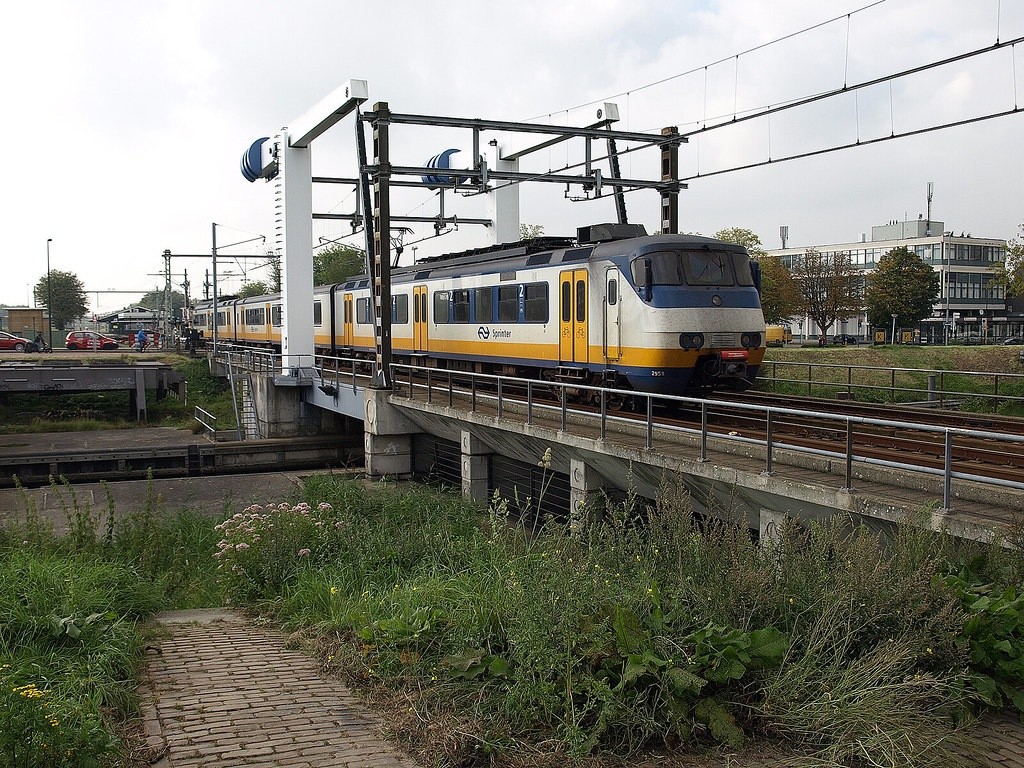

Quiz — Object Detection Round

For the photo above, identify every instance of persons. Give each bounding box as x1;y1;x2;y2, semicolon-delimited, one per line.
138;329;147;352
86;334;91;338
34;332;44;352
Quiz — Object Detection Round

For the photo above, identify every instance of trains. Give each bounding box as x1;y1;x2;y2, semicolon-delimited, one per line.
176;226;764;413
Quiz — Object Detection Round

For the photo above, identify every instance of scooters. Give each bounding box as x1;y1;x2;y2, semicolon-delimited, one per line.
25;341;52;353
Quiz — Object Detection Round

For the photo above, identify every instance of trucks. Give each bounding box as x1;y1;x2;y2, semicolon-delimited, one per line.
763;321;792;344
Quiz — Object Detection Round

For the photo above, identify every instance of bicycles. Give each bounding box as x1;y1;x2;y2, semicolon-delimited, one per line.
129;341;158;354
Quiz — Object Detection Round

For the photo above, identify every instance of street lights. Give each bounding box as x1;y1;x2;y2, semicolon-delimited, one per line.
46;239;53;348
891;314;898;344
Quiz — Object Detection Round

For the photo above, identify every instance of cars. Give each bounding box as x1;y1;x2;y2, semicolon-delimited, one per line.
1003;339;1024;345
0;332;33;352
833;334;857;346
64;331;120;350
